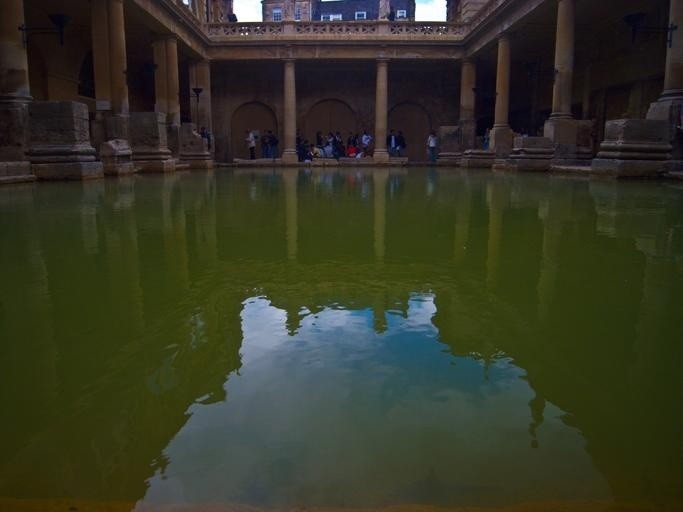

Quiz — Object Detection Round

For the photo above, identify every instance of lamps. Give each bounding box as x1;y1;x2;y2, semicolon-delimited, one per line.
470;86;499;99
178;87;202;104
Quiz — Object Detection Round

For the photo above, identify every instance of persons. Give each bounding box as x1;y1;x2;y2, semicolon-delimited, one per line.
296;127;373;162
396;129;407;157
386;6;395;32
225;8;237;35
193;127;211;151
386;128;400;157
483;128;490;150
249;166;439;208
427;132;439;162
245;128;279;160
